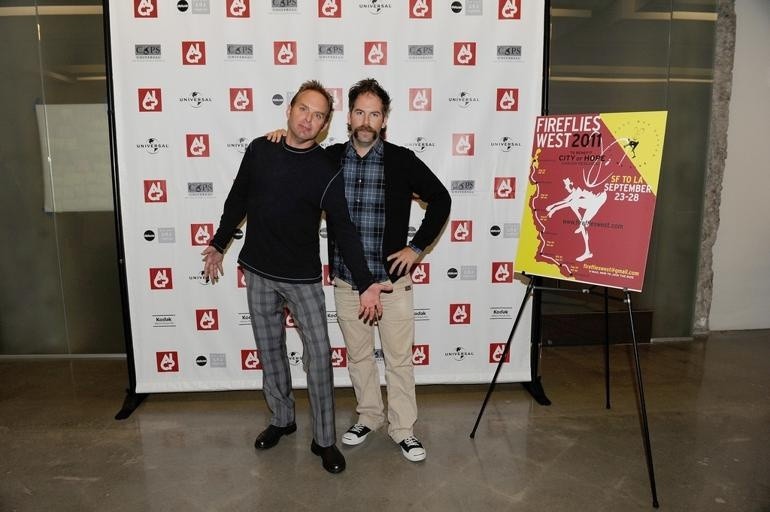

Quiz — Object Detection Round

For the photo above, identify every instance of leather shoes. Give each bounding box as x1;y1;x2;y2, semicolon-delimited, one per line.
255;422;297;450
311;438;347;473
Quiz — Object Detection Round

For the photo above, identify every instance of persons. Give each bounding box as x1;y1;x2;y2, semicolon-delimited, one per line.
201;77;392;471
266;80;451;460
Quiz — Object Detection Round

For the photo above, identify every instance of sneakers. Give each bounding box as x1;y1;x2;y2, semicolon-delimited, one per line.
399;434;426;462
341;423;373;446
